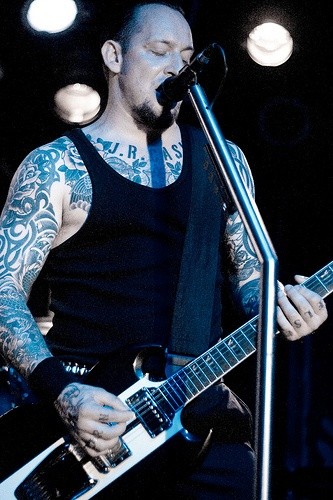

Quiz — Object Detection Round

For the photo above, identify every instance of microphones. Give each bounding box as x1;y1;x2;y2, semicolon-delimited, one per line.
155;42;217;109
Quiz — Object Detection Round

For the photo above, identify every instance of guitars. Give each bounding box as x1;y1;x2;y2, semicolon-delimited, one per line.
0;260;333;500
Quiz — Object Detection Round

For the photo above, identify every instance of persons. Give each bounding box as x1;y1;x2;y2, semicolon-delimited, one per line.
0;0;327;500
